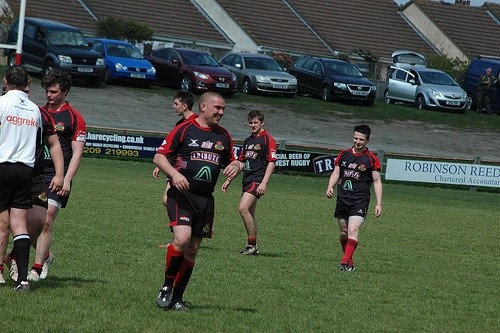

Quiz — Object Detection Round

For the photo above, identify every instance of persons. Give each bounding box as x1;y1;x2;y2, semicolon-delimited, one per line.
0;64;64;292
476;68;498;115
152;92;240;311
221;110;276;254
26;69;85;282
327;125;383;272
153;90;199;248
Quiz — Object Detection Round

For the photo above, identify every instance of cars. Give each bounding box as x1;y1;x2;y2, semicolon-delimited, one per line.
144;47;238;99
212;52;298;98
383;50;469;115
77;37;157;89
289;55;377;106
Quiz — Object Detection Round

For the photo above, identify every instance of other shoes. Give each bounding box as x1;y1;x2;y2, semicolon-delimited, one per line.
0;266;6;283
13;280;30;292
39;252;55;279
4;252;18;282
337;264;355;272
27;269;40;282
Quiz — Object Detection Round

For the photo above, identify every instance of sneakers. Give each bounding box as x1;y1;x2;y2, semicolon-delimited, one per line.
170;299;194;312
239;242;260;255
155;286;174;308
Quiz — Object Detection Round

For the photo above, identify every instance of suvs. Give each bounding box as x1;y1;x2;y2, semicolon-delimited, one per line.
4;17;106;87
462;54;500;115
